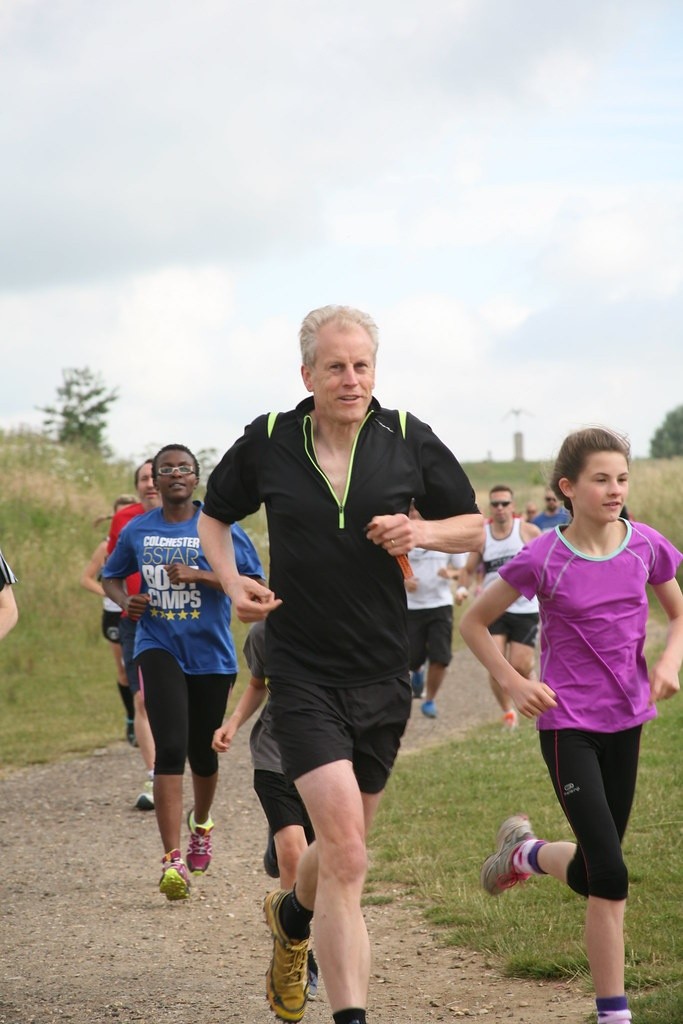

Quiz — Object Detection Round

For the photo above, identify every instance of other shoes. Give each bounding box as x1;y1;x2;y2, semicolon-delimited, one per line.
411;671;425;697
134;780;155;810
125;716;138;747
500;710;518;733
421;701;437;716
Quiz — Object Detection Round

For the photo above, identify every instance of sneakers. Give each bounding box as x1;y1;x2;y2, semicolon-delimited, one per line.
263;887;318;1024
186;807;214;876
264;826;280;878
481;816;536;896
159;849;191;901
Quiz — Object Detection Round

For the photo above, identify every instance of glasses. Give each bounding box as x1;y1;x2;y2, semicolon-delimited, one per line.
490;501;511;507
544;497;557;502
155;466;196;476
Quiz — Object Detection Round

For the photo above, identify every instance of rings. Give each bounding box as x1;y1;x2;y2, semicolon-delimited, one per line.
390;539;398;549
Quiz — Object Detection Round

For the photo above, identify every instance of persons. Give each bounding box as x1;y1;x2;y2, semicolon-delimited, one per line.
195;304;487;1023
0;547;19;642
407;482;631;734
78;443;269;904
457;424;683;1024
211;592;320;1001
